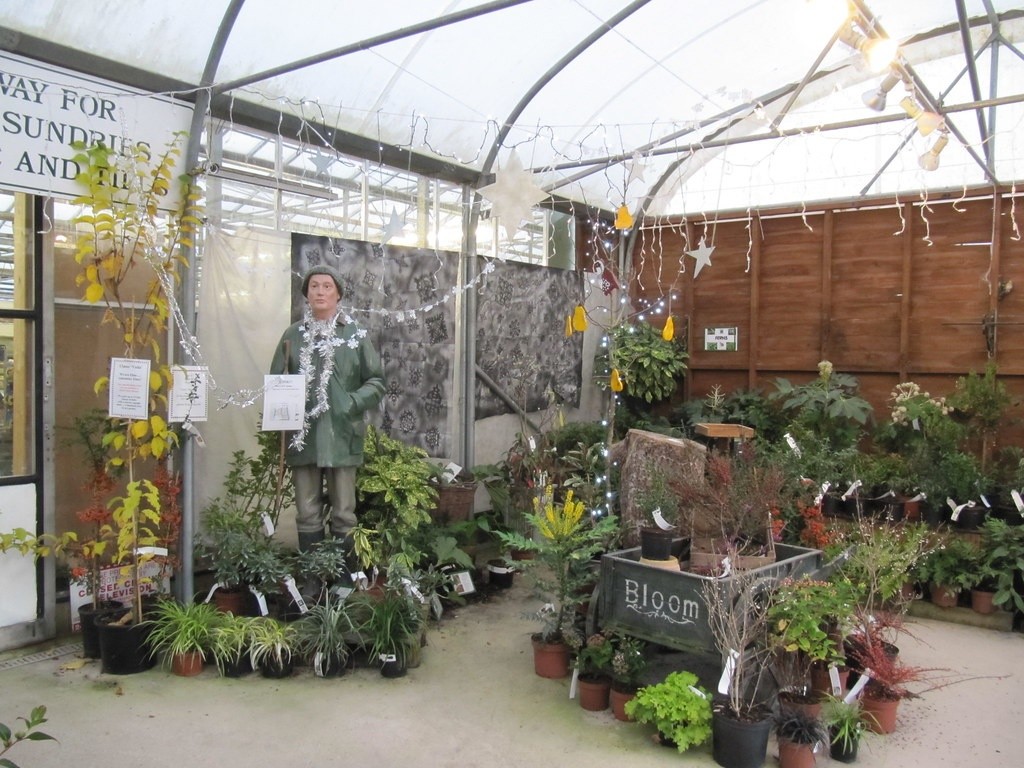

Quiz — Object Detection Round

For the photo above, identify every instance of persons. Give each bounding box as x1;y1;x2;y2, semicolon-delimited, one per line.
270;265;388;605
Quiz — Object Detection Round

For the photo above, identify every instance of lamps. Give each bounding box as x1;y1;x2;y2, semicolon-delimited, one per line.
900;95;945;136
862;69;903;112
918;135;949;172
839;25;897;66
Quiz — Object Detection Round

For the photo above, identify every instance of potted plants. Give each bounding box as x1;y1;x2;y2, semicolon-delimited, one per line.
0;131;1024;768
